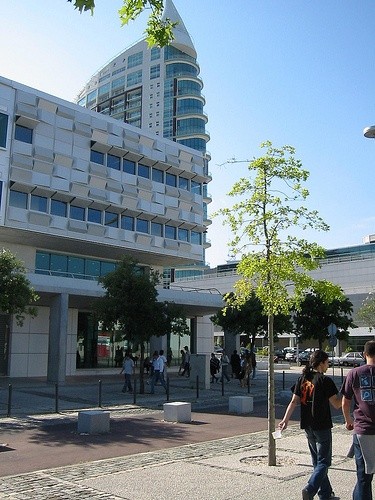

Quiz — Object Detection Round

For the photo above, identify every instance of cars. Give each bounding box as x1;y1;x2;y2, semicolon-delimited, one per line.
215;341;368;370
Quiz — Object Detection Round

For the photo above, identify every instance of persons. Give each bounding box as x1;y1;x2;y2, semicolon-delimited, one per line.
210;352;220;383
231;350;257;389
144;350;170;394
120;355;135;394
220;349;231;384
341;341;375;500
178;346;193;377
279;350;343;500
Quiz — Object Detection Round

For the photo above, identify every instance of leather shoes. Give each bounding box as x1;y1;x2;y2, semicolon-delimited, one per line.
318;493;340;500
302;488;313;500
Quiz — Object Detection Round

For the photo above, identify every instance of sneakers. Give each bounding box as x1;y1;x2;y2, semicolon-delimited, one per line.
217;381;223;385
226;378;232;384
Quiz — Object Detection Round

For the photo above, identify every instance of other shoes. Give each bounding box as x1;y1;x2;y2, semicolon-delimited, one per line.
173;370;190;377
